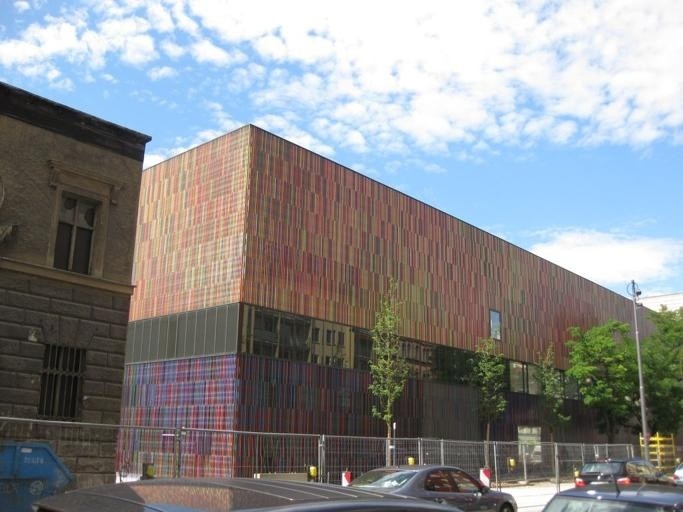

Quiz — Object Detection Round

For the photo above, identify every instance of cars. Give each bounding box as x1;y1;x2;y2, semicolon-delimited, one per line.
538;458;683;511
345;462;520;512
29;475;463;511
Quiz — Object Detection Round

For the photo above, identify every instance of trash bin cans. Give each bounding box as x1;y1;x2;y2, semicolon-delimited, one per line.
0;442;72;512
